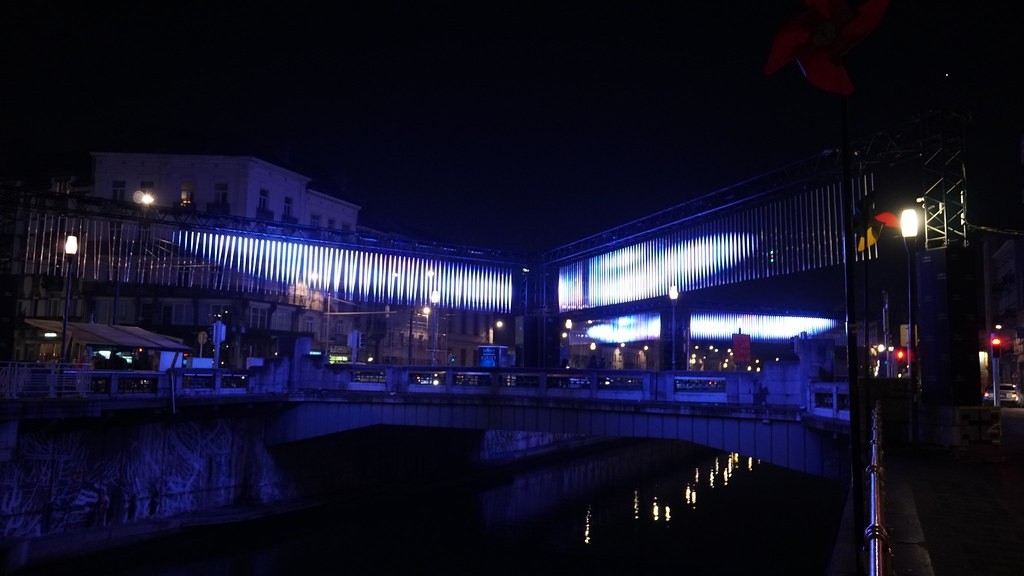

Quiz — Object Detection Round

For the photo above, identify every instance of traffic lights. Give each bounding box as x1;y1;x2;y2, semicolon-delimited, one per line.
209;321;216;346
993;337;1001;358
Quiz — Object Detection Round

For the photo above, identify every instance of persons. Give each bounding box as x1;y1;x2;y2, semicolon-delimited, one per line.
586;355;598;369
558;358;571;389
599;357;606;368
90;352;128;383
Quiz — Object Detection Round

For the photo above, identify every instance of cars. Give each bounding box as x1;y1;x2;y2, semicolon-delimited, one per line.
984;384;1021;406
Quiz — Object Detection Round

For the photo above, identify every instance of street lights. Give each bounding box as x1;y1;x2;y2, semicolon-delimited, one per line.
432;291;440;365
900;209;921;443
669;286;678;370
61;235;78;363
996;325;1001;383
566;319;572;369
